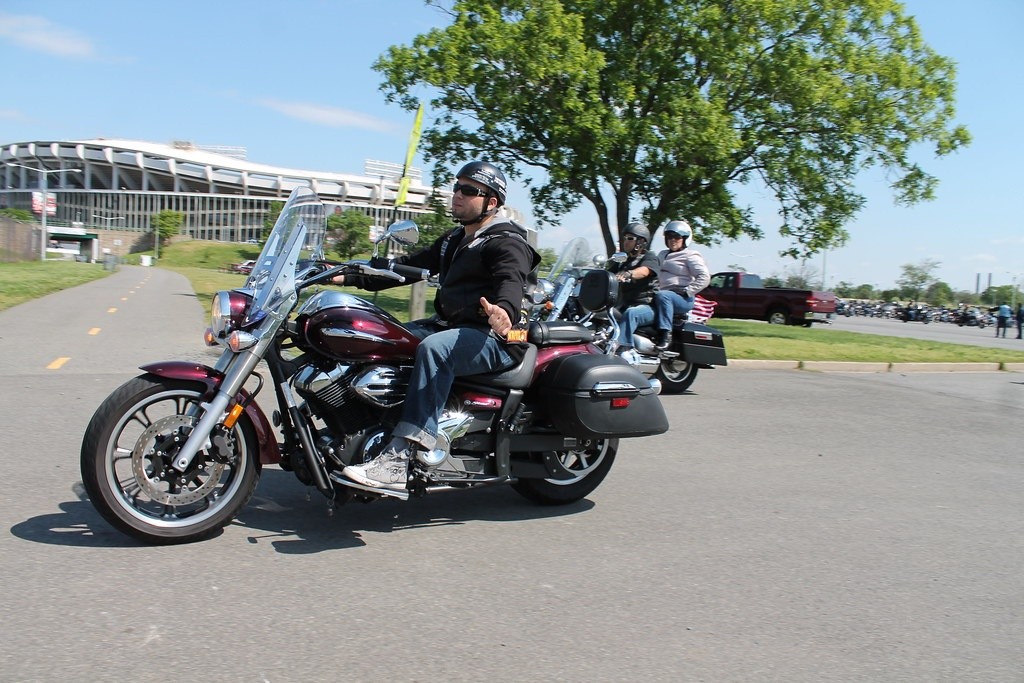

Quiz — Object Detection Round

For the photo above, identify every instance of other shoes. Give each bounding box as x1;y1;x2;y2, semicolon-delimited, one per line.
619;348;641;367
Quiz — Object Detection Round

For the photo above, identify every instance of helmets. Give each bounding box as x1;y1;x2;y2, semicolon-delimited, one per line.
622;223;651;245
455;161;507;198
663;221;692;248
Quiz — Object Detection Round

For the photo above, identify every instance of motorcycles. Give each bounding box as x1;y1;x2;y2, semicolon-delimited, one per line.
835;299;1016;329
518;237;728;393
80;185;670;546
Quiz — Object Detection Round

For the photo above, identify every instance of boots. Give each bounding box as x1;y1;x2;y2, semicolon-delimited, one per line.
654;331;673;350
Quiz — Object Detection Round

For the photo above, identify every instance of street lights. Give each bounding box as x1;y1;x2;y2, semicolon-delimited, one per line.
1005;270;1024;308
6;162;82;261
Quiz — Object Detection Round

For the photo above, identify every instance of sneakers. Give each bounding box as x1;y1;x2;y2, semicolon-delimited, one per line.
343;445;410;489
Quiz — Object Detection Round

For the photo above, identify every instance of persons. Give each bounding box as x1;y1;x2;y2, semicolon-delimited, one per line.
988;302;1015;337
1011;303;1024;339
654;221;711;352
321;162;542;493
848;300;983;323
604;224;662;354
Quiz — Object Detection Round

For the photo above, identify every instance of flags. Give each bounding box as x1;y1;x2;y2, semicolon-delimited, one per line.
689;295;718;325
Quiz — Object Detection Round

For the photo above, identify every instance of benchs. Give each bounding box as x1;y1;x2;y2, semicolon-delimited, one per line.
216;266;241;272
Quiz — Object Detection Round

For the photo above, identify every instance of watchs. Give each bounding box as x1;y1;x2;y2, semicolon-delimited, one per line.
627;270;632;279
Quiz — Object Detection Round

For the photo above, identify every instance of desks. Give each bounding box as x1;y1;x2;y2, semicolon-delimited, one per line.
224;261;241;274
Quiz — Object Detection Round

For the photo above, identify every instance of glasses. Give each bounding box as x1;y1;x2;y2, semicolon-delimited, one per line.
623;236;641;241
666;234;684;240
453;182;488;198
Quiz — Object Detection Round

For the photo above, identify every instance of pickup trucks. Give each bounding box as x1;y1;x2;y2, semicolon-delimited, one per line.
696;272;835;325
